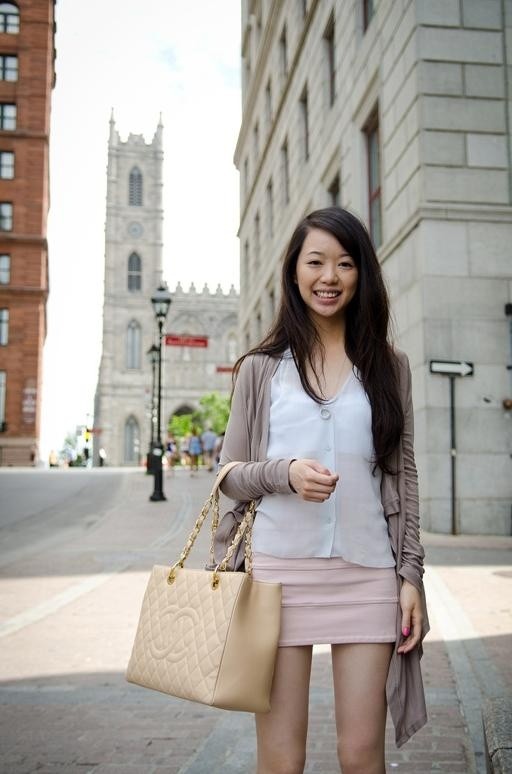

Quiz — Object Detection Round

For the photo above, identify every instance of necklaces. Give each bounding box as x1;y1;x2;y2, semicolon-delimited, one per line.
314;351;346;419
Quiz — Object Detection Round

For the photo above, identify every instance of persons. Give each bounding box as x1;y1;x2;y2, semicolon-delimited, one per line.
214;206;433;774
165;426;224;476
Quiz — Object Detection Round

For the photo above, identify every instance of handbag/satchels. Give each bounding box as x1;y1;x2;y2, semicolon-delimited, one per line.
120;459;291;715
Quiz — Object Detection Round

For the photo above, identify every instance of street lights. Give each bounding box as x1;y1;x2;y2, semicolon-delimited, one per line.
147;285;172;502
